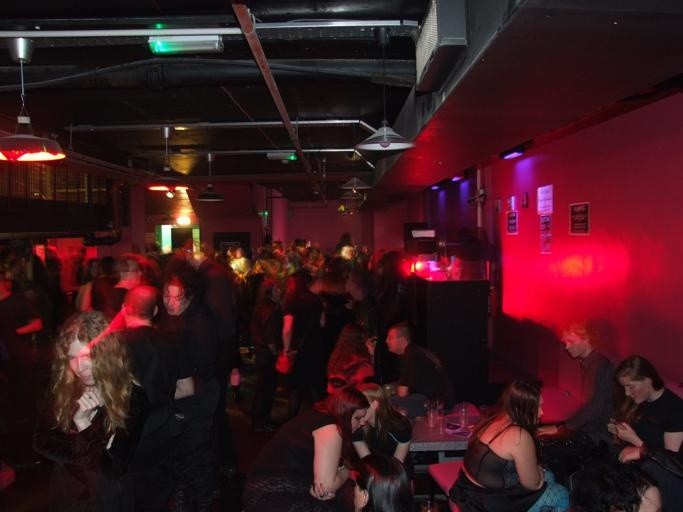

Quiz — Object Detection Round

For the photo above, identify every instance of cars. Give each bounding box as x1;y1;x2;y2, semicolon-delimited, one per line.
384;383;392;403
424;396;470;440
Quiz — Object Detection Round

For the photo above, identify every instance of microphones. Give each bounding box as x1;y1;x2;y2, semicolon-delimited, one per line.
537;379;592;425
428;460;467;512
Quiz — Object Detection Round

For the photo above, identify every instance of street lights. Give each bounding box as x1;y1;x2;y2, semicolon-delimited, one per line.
641;448;683;511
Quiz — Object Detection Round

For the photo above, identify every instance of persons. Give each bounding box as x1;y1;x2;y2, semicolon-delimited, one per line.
241;383;370;509
32;310;151;509
0;232;454;512
455;375;568;509
539;330;683;512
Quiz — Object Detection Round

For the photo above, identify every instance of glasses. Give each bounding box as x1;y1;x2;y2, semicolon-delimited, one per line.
339;153;373;194
0;37;66;161
195;153;226;201
355;26;416;151
148;127;190;191
339;190;368;200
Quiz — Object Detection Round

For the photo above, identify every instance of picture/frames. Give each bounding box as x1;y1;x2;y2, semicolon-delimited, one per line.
408;416;480;501
232;346;274;401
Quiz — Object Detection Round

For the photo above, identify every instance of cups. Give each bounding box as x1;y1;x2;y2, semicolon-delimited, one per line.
368;336;378;346
